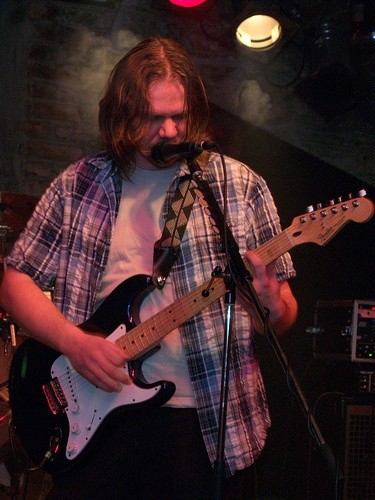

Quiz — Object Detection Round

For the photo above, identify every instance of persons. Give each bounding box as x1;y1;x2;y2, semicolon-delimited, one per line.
0;36;300;500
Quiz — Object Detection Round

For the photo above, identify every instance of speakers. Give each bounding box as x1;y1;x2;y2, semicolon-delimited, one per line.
323;396;375;500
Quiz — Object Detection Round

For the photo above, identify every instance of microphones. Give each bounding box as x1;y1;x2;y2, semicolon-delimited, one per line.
152;140;214;169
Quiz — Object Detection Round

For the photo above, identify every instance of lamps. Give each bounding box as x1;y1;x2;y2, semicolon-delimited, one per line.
218;1;298;66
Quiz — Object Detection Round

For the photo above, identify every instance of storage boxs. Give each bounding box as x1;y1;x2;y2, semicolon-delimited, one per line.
312;299;375;362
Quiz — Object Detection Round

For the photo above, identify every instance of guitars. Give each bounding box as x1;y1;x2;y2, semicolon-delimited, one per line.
8;187;374;474
